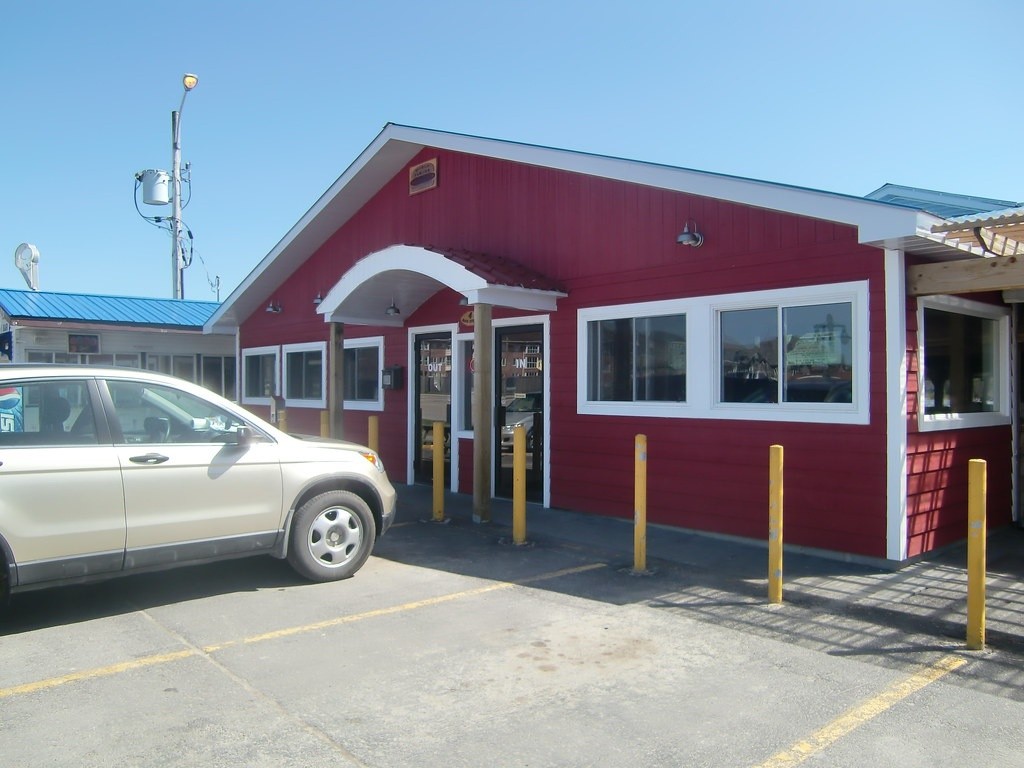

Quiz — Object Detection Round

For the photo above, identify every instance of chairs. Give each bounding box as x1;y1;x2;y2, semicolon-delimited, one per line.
46;396;71;431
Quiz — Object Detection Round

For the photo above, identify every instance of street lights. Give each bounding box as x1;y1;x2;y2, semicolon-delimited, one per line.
171;72;197;300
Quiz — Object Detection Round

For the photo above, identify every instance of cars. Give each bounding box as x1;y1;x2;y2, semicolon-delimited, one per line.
500;391;544;454
0;362;400;634
613;355;989;415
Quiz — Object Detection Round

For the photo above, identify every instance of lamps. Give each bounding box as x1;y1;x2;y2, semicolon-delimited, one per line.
670;215;704;248
458;296;468;309
264;298;280;314
312;286;327;305
384;290;400;318
